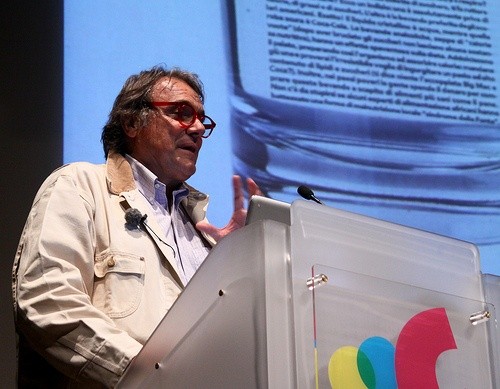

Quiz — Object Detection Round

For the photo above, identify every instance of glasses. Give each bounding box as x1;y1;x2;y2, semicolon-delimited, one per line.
144;101;216;139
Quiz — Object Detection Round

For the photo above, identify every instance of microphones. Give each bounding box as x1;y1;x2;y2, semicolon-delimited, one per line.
298;185;322;204
124;208;147;227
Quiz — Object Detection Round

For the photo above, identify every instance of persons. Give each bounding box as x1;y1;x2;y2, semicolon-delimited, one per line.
10;67;264;389
